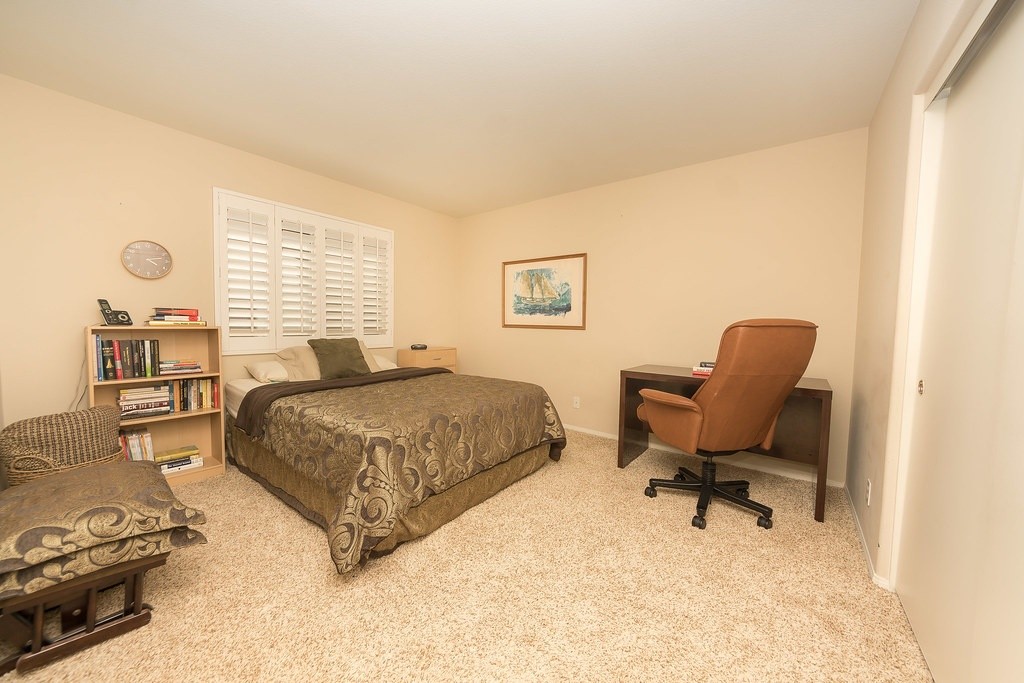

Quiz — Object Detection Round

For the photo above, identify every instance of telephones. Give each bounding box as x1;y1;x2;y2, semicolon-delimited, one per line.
97;299;134;325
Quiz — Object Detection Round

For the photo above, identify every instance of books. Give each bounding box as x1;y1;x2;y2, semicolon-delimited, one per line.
116;379;219;421
119;426;204;475
91;334;204;383
692;360;717;376
144;307;208;327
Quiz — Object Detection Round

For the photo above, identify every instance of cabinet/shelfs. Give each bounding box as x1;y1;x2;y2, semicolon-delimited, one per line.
397;347;458;375
84;324;226;488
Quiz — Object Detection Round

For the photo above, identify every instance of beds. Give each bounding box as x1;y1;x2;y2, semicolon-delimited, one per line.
224;366;567;575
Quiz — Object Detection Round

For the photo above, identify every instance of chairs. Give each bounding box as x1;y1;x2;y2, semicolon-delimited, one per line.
637;319;819;530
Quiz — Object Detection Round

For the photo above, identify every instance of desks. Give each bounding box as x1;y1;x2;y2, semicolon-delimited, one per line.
617;364;833;523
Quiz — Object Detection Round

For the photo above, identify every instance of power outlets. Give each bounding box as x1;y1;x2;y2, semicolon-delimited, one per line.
864;479;871;507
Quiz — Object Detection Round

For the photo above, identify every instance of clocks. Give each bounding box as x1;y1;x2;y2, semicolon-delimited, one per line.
121;240;174;279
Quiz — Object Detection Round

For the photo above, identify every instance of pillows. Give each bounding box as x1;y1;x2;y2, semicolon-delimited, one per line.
372;355;397;371
0;460;208;601
306;337;373;380
244;361;289;383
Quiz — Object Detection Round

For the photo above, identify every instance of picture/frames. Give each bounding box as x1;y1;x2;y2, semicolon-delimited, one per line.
502;253;587;331
